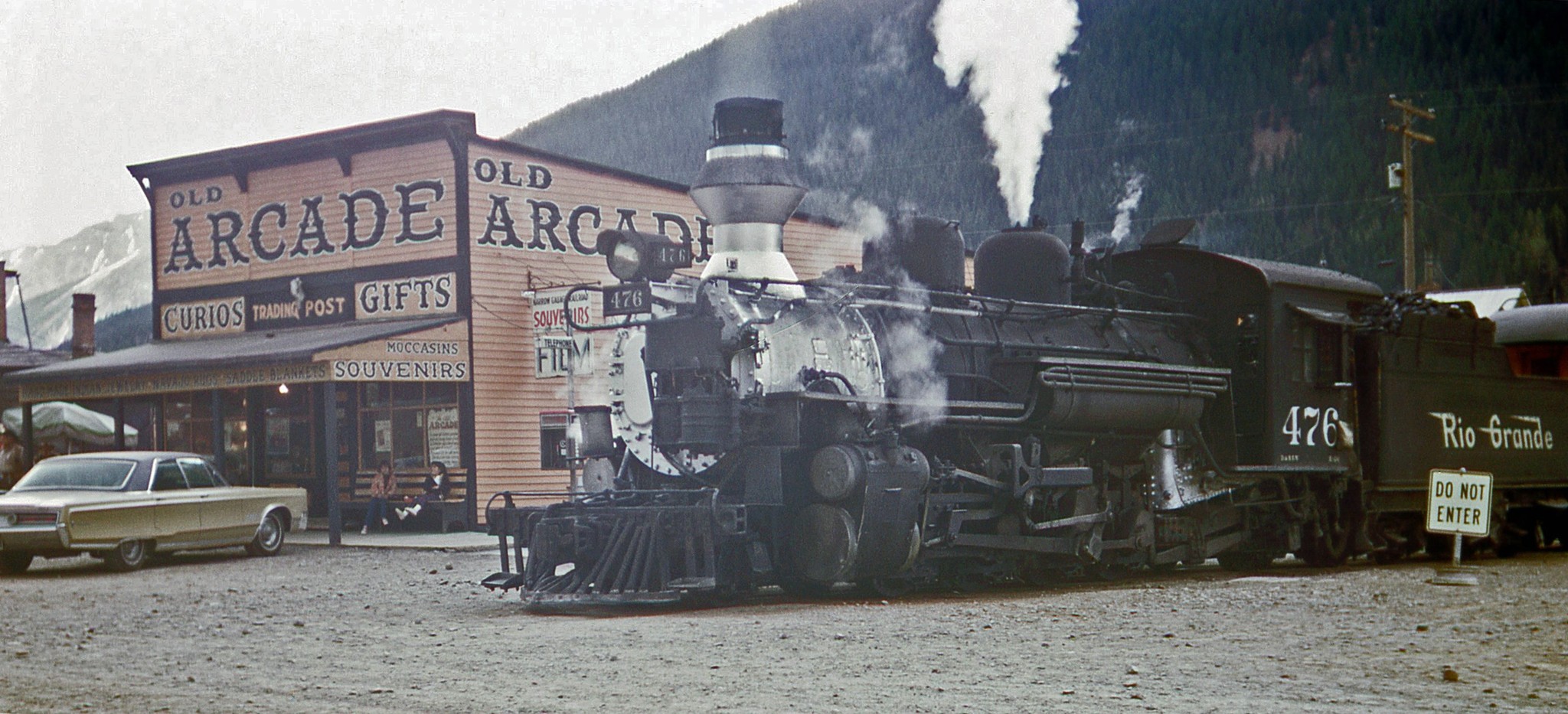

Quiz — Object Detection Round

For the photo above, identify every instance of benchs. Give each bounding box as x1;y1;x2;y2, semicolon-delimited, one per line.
339;467;471;534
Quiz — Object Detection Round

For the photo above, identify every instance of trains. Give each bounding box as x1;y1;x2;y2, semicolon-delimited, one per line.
471;91;1567;609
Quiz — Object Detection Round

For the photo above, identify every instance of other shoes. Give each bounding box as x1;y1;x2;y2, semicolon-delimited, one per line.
381;517;388;525
361;525;369;534
394;508;404;520
404;507;417;517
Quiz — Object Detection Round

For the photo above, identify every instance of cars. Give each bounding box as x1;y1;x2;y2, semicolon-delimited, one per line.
1;450;312;573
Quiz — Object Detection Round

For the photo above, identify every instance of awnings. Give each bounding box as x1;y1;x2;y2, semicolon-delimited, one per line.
3;314;471;387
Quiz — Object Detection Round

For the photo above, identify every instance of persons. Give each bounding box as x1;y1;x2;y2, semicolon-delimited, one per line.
360;460;399;535
393;461;451;520
0;431;24;475
33;441;61;469
